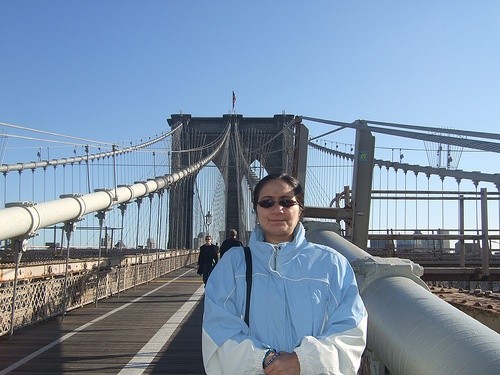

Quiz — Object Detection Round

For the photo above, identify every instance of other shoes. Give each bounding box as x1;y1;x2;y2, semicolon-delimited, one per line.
204;285;206;288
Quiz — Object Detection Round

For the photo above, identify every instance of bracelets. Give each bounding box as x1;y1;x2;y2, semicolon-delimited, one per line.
262;347;279;369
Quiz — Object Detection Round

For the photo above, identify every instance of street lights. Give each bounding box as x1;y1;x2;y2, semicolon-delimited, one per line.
204;211;213;236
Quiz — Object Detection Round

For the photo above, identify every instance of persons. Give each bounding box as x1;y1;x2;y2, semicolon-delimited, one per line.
196;228;245;290
202;171;369;375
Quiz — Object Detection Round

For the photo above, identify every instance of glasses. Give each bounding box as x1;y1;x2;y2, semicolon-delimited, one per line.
205;239;210;240
255;198;304;208
